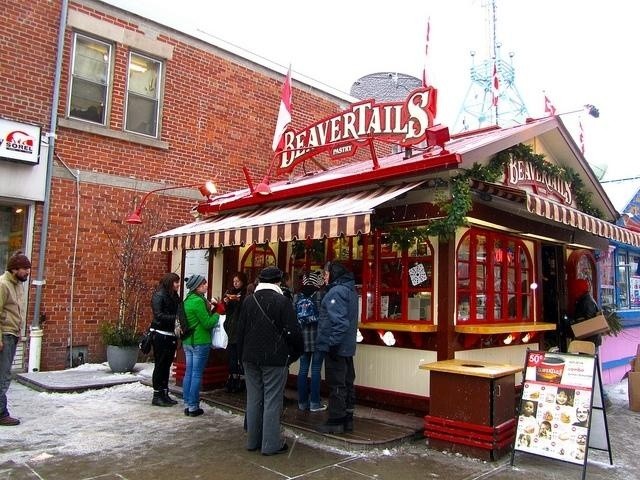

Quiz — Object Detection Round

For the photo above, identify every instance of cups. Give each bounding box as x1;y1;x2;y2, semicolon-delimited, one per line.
536;357;565;384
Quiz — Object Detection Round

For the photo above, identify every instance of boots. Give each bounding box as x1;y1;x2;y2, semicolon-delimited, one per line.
152;391;172;406
162;388;178;404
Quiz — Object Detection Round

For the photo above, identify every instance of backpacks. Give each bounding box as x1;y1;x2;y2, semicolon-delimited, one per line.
178;296;201;340
296;292;318;325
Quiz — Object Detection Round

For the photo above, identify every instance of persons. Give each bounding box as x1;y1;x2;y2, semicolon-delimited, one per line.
518;434;530;448
536;421;551;440
520;401;535;418
0;253;34;427
556;277;613;412
221;262;361;457
557;386;572;407
418;245;427;256
149;273;181;406
570;407;589;428
184;274;221;417
574;445;585;460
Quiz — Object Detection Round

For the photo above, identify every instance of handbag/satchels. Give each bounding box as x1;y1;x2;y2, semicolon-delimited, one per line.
211;315;228;350
139;332;155;354
273;330;303;364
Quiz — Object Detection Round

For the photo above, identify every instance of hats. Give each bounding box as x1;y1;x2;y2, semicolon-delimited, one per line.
259;266;283;282
185;274;205;290
303;274;318;285
7;251;30;270
160;273;179;286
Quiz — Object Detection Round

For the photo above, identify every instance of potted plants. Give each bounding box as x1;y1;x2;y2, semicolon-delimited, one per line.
99;319;145;373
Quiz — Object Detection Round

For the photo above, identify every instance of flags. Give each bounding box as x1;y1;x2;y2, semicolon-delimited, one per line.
492;62;501;106
543;94;556;117
272;67;293;153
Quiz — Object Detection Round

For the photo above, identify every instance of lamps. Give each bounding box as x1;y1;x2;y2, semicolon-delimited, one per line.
197;181;218;202
125;166;292;225
250;137;430;198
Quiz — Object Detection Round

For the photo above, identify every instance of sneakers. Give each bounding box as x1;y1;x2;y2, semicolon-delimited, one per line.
248;447;262;451
310;405;327;411
343;413;353;433
319;418;343;433
1;416;20;425
185;408;189;416
263;444;288;455
189;409;203;416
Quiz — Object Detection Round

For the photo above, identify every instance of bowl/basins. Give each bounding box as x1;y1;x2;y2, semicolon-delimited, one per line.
227;294;241;299
